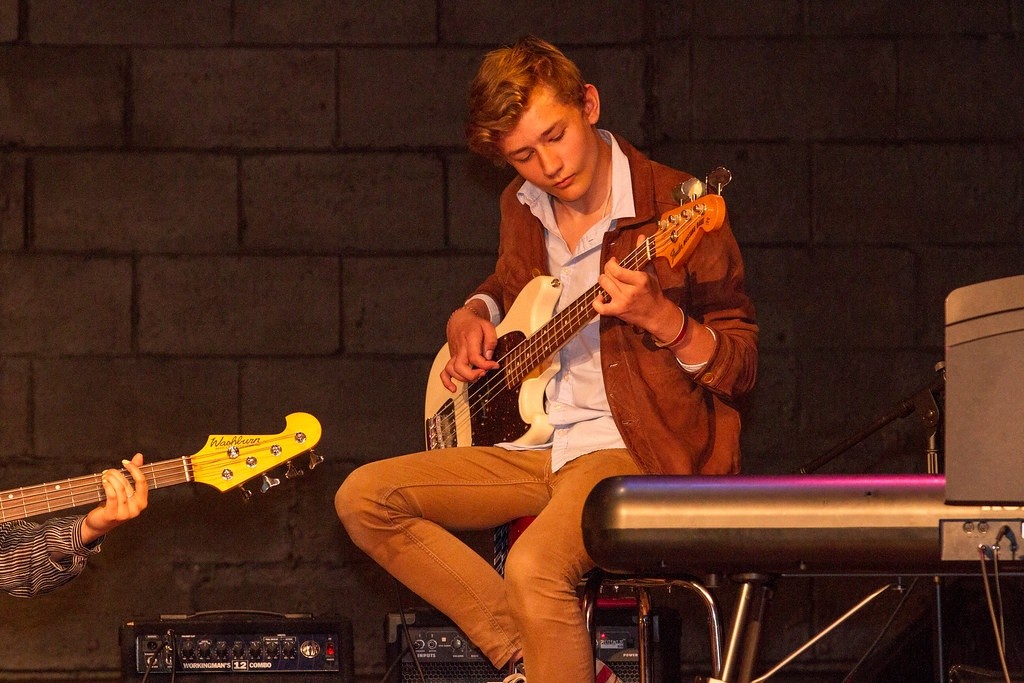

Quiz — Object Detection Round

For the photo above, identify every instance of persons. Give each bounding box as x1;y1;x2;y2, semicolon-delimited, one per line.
335;40;760;683
0;452;149;599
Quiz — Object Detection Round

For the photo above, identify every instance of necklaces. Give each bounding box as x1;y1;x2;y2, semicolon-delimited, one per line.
596;188;614;220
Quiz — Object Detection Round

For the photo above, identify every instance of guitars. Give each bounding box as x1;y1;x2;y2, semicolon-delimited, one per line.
425;176;726;449
0;412;323;523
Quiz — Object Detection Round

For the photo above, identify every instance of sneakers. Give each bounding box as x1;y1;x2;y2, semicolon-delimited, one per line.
503;662;527;683
595;659;623;683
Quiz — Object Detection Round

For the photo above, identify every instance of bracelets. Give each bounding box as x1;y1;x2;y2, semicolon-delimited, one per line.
444;304;479;339
650;306;688;351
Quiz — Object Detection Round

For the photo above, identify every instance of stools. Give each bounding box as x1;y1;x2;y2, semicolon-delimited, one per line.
509;514;725;683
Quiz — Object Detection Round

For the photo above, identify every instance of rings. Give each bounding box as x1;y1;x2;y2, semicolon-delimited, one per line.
125;491;136;499
